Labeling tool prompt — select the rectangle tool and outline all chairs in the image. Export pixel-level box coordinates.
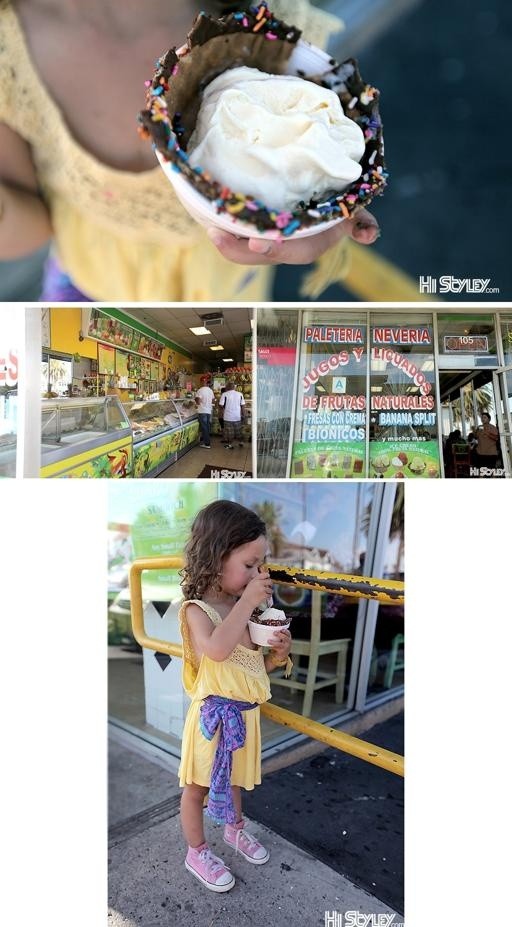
[263,589,351,719]
[452,442,471,477]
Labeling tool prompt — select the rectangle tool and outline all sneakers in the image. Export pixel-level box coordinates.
[222,819,270,865]
[185,841,236,893]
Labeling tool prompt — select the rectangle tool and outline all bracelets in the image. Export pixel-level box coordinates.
[266,649,289,667]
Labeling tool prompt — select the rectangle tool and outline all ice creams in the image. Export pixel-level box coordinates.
[250,608,292,626]
[138,6,387,238]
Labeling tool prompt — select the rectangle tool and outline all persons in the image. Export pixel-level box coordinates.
[1,0,384,302]
[216,387,231,444]
[177,498,294,894]
[194,375,217,449]
[472,412,500,478]
[442,429,478,475]
[218,383,247,450]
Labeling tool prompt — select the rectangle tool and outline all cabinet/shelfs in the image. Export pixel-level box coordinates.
[41,371,252,479]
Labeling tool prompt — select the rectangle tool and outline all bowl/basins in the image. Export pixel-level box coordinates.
[371,459,437,478]
